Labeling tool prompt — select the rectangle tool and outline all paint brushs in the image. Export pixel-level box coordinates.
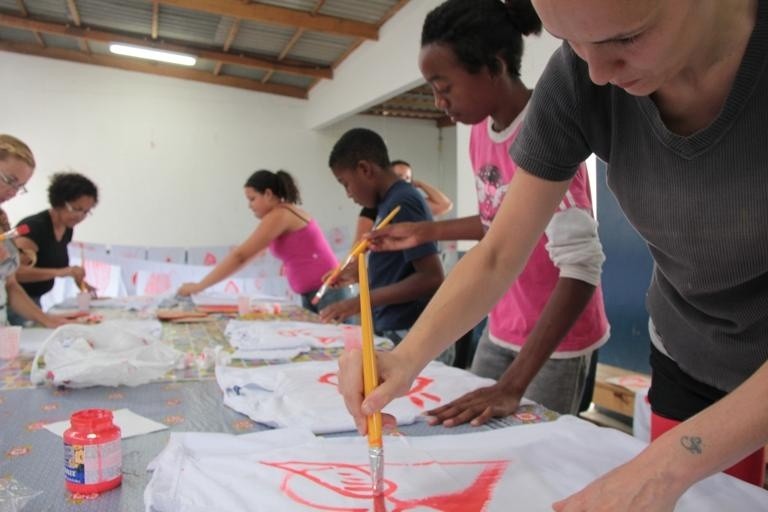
[0,223,30,242]
[357,253,388,512]
[80,249,87,293]
[311,204,402,306]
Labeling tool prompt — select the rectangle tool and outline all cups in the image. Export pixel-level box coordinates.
[2,325,21,360]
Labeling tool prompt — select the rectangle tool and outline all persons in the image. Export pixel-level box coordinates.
[336,0,768,512]
[359,0,611,431]
[6,171,100,328]
[0,133,97,329]
[317,127,456,367]
[350,159,452,259]
[175,168,357,326]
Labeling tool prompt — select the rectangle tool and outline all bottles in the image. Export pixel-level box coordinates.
[62,407,124,495]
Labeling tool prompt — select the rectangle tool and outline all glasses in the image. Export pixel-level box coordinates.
[0,169,28,196]
[65,202,93,218]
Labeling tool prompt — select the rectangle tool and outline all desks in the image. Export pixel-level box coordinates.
[0,293,768,512]
